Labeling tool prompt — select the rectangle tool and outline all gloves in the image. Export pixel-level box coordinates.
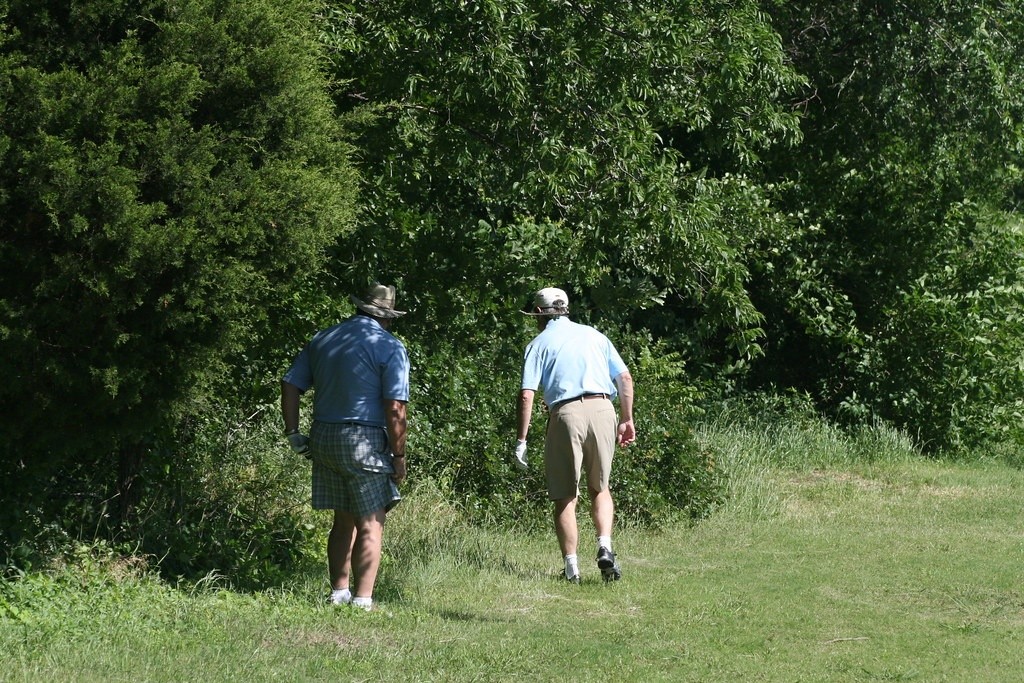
[514,438,528,470]
[285,427,312,460]
[391,454,407,487]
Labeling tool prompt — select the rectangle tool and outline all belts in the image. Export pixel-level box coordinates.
[560,393,611,402]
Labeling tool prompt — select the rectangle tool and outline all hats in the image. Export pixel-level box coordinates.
[351,280,407,318]
[531,287,569,313]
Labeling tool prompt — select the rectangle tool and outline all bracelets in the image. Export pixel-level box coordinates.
[391,454,405,458]
[283,427,299,436]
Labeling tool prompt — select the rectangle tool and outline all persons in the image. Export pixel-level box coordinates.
[514,287,636,583]
[280,284,411,617]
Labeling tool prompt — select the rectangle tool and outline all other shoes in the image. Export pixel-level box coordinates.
[348,602,393,620]
[330,593,354,608]
[595,546,621,583]
[558,569,581,586]
